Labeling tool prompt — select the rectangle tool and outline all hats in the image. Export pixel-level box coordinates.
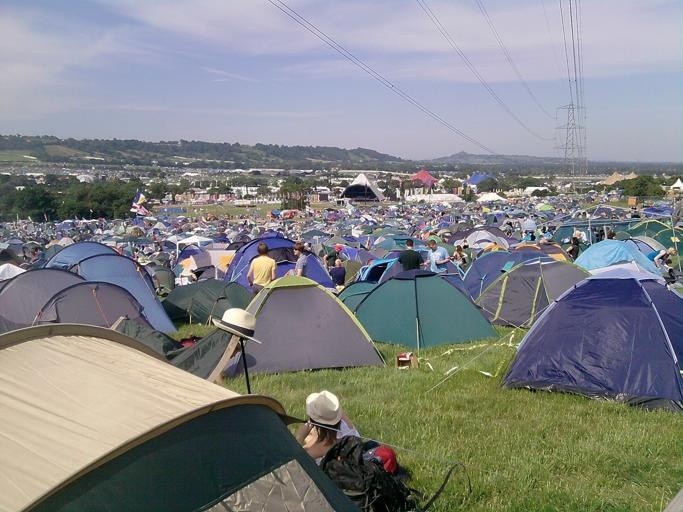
[211,307,262,345]
[305,390,342,426]
[335,245,342,251]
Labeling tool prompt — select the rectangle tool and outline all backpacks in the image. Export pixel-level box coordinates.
[319,435,421,512]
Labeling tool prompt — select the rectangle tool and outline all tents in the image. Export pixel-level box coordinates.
[0,188,682,415]
[0,323,366,512]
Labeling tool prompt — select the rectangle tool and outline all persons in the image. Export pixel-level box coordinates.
[296,390,361,467]
